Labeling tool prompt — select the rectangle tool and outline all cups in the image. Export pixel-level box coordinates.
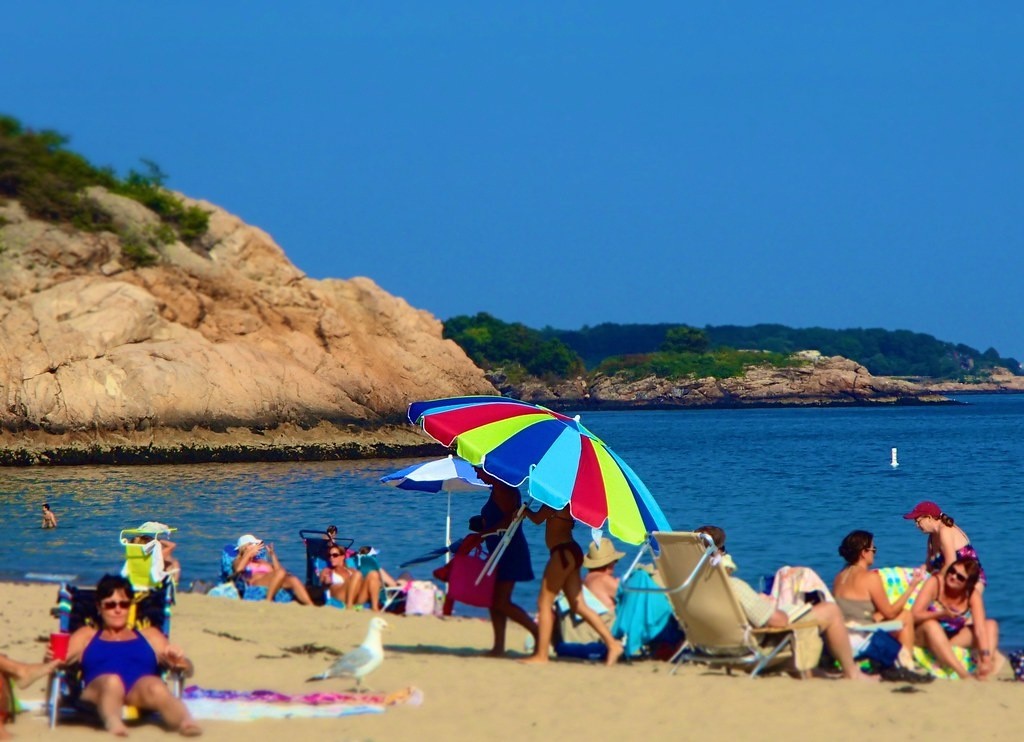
[50,633,72,663]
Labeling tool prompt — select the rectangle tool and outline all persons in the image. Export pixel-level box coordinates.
[360,546,407,588]
[904,502,987,624]
[0,654,60,688]
[520,502,624,667]
[831,530,936,683]
[43,573,202,736]
[911,555,998,681]
[468,464,539,657]
[583,538,627,610]
[233,533,316,606]
[322,526,338,546]
[131,534,180,583]
[41,503,57,528]
[329,547,382,613]
[692,526,881,683]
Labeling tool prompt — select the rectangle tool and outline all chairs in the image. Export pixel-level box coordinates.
[360,556,407,613]
[50,577,182,734]
[222,543,289,600]
[777,564,904,668]
[876,567,976,679]
[315,555,367,609]
[620,530,808,682]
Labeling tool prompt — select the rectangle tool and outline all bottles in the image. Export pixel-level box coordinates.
[436,590,446,616]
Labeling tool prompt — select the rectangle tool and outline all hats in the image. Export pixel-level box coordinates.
[583,536,626,570]
[902,501,942,520]
[359,547,382,560]
[234,533,263,552]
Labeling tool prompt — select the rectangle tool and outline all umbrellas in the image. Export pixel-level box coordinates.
[399,538,466,569]
[406,395,672,587]
[378,454,492,595]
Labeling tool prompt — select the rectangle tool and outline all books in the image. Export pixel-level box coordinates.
[778,600,813,625]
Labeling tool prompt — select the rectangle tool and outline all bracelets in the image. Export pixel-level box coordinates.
[979,650,989,656]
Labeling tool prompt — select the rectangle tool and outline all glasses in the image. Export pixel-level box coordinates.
[718,546,726,552]
[327,553,344,558]
[948,567,971,585]
[865,547,877,553]
[104,601,136,610]
